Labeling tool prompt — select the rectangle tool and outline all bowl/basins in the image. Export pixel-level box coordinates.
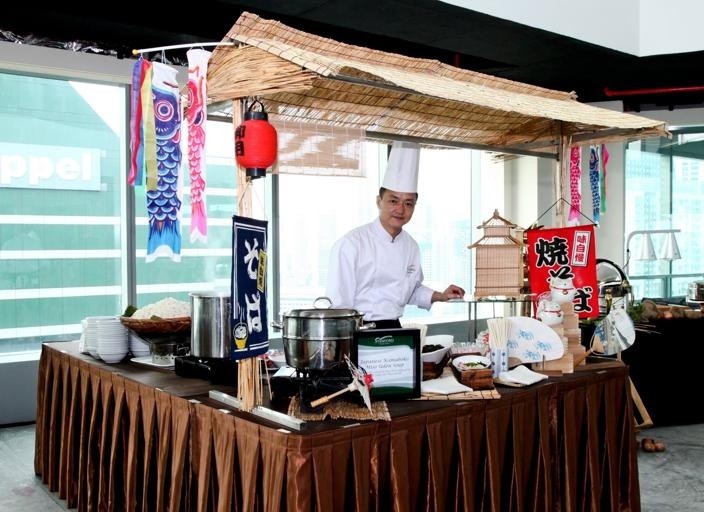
[189,290,237,359]
[449,341,490,360]
[117,315,191,341]
[85,312,151,363]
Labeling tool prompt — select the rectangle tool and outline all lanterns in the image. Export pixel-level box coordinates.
[233,109,281,181]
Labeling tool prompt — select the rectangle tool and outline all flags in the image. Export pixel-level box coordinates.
[527,225,598,319]
[228,215,269,362]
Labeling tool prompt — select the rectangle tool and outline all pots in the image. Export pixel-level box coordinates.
[270,297,376,373]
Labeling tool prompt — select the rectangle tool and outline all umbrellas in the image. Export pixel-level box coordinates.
[309,350,376,419]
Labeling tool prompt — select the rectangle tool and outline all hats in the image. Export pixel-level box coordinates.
[381,141,420,194]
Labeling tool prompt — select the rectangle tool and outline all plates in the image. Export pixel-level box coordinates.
[130,355,181,371]
[490,317,565,363]
[452,356,490,372]
[422,335,454,363]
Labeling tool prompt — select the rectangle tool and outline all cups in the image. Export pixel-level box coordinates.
[490,347,509,377]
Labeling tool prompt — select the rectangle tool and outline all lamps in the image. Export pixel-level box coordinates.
[626,228,682,278]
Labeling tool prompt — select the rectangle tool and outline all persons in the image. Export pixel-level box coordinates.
[335,185,468,330]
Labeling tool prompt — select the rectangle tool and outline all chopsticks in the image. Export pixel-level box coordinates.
[487,318,513,348]
